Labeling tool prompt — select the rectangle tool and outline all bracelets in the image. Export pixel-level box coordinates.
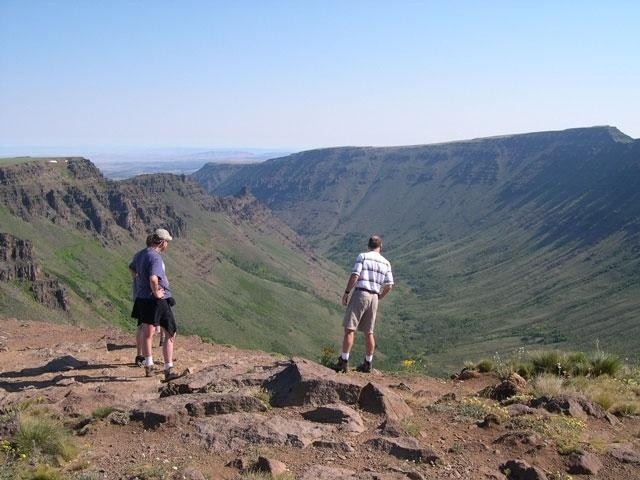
[345,289,350,294]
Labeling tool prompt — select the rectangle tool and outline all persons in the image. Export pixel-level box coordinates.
[336,235,394,372]
[128,229,186,381]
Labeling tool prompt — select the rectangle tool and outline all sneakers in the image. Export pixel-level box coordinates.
[160,340,165,346]
[164,367,181,380]
[135,355,164,377]
[357,361,371,373]
[154,229,173,241]
[331,357,349,373]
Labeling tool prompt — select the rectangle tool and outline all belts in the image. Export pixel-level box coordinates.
[355,287,376,294]
